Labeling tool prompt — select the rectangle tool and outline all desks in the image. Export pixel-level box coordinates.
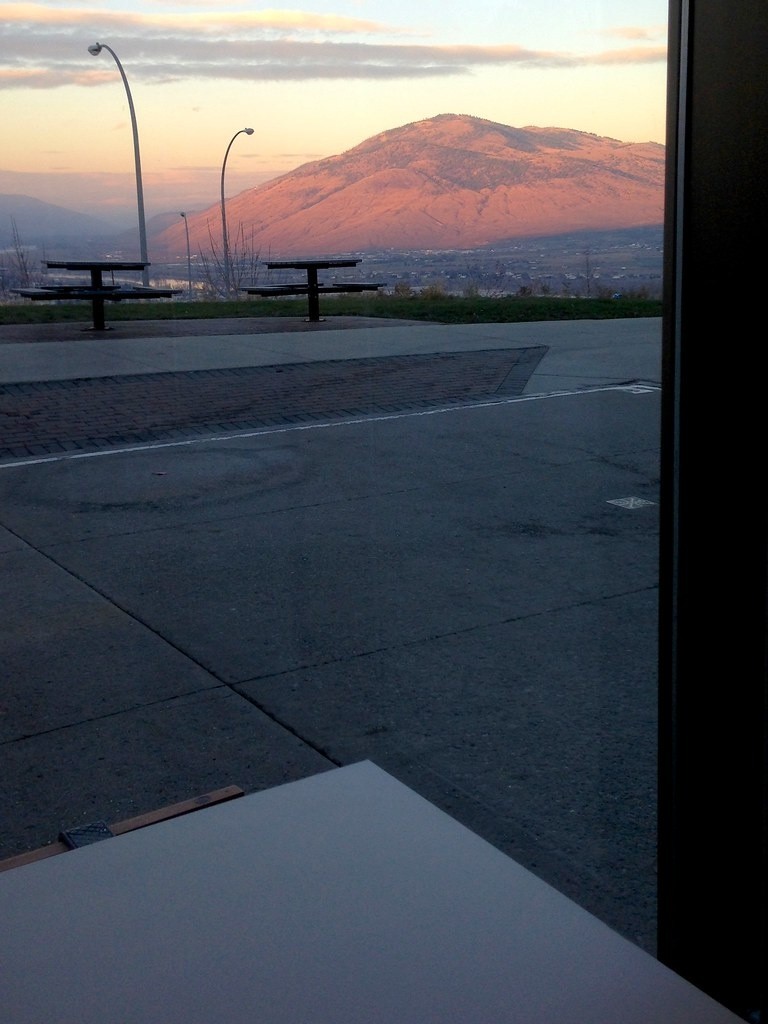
[20,260,173,331]
[245,259,379,322]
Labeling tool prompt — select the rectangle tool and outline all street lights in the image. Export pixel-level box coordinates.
[221,128,254,301]
[180,212,192,302]
[88,42,150,303]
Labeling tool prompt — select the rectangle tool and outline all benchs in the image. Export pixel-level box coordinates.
[333,281,387,288]
[258,282,325,289]
[10,288,57,295]
[133,285,183,295]
[237,285,288,292]
[37,285,121,291]
